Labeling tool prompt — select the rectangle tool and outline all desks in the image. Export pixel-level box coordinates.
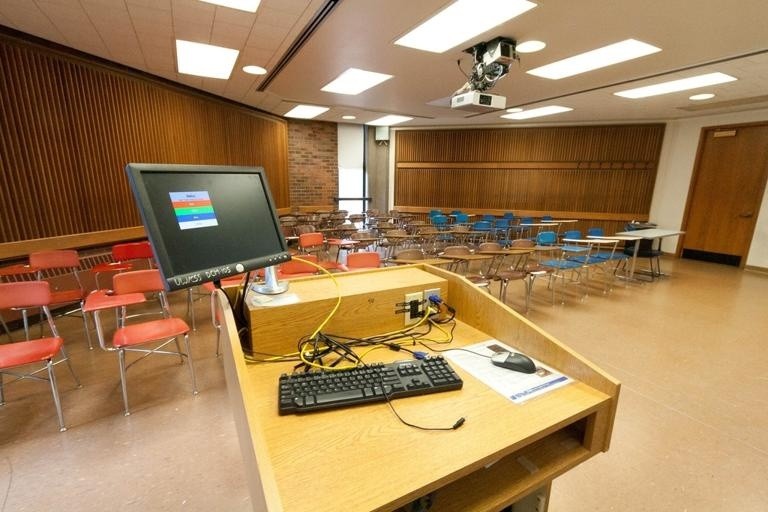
[90,260,134,273]
[1,263,40,276]
[83,289,147,312]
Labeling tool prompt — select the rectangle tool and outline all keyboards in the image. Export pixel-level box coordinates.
[279,356,463,415]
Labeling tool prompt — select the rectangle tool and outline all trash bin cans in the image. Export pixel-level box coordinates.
[623,222,658,257]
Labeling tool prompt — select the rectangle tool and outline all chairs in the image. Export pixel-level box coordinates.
[187,203,685,316]
[1,280,84,434]
[11,248,94,351]
[94,269,200,417]
[94,240,156,291]
[203,279,248,356]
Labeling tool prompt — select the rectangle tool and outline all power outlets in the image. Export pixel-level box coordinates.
[423,288,441,321]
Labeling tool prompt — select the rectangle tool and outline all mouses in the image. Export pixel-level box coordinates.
[492,352,536,374]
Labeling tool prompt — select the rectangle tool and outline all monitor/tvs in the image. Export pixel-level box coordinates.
[125,163,291,293]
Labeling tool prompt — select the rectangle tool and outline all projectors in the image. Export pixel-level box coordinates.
[451,92,506,113]
